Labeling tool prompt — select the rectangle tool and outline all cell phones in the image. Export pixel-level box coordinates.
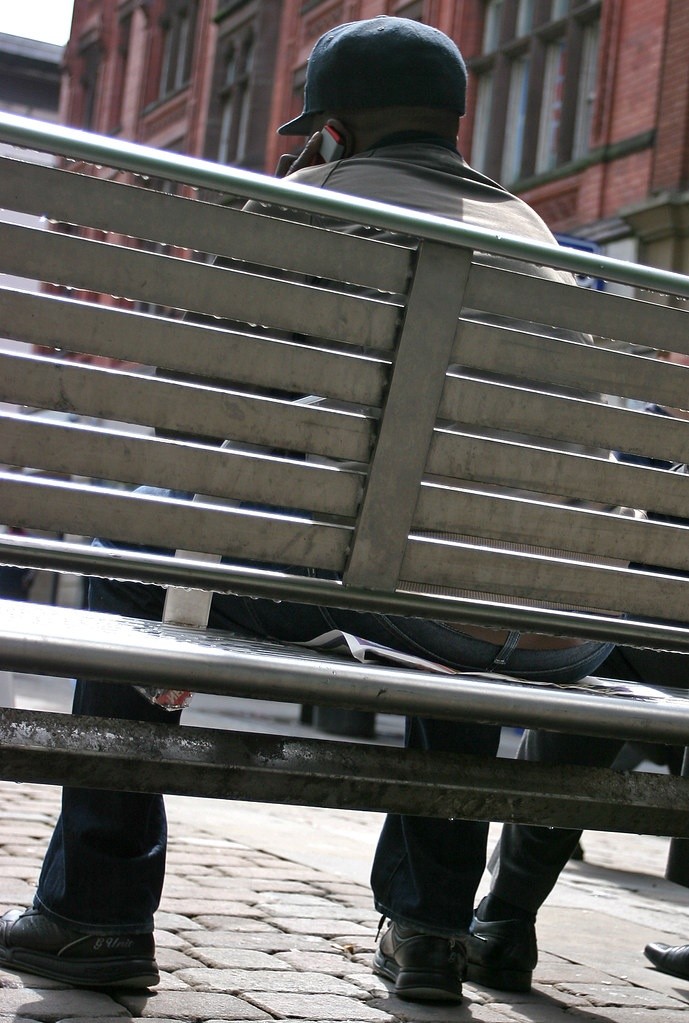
[311,126,344,167]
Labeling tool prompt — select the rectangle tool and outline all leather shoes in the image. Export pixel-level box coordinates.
[644,940,689,977]
[459,895,538,988]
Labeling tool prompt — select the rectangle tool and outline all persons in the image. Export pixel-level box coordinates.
[461,462,689,991]
[0,15,617,1005]
[615,351,688,469]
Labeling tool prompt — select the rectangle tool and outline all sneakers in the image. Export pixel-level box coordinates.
[374,911,464,1005]
[0,902,161,987]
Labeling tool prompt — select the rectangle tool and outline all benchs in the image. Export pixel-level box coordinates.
[0,114,689,837]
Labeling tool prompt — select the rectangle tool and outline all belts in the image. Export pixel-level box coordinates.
[439,617,590,650]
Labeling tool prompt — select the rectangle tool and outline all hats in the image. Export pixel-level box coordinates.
[275,15,467,135]
